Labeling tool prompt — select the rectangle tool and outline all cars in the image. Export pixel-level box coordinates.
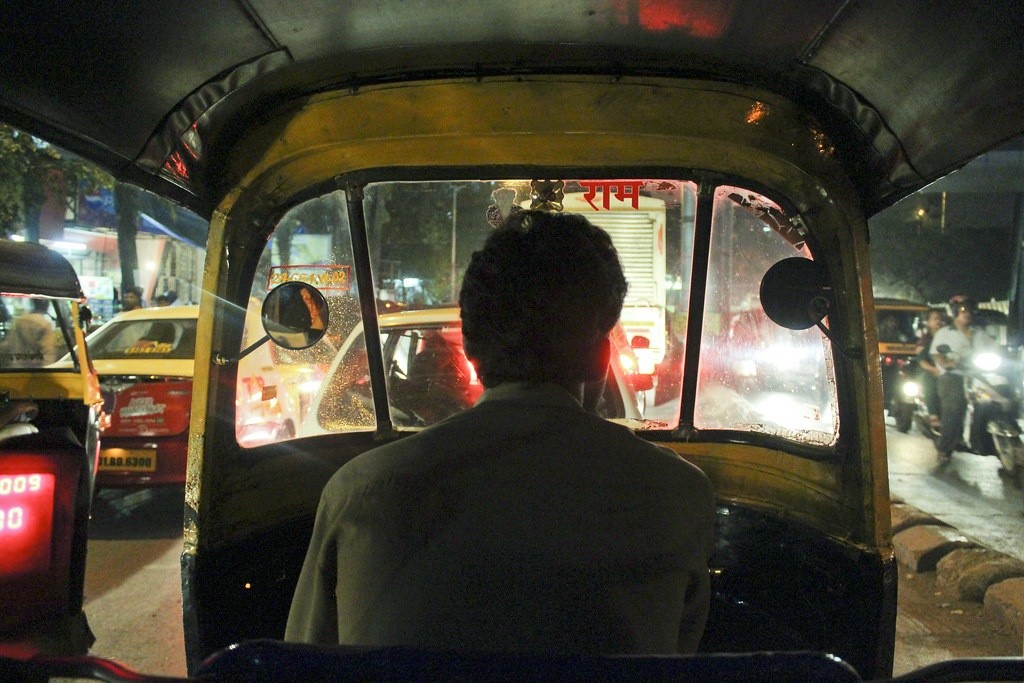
[43,304,323,486]
[311,307,641,432]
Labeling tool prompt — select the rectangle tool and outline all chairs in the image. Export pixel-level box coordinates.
[138,323,178,344]
[406,348,472,392]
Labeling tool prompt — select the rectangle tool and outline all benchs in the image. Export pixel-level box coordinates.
[201,637,860,683]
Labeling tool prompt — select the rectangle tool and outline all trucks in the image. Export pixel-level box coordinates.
[515,191,674,416]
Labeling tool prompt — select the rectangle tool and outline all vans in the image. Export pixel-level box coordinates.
[1,234,99,659]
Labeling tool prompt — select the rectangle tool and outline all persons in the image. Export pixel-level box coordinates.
[284,208,713,660]
[0,287,184,366]
[874,293,1005,470]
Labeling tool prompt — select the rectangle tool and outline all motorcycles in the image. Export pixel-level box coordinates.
[868,297,939,433]
[914,330,1024,480]
